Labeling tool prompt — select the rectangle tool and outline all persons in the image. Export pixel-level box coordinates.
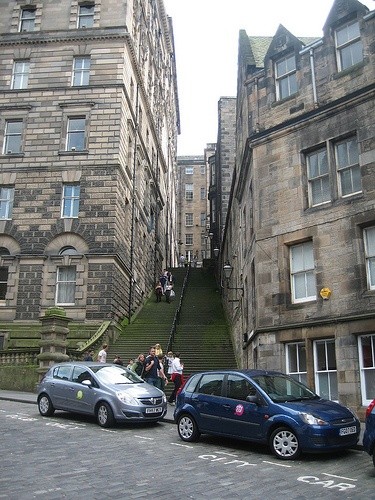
[179,254,188,268]
[193,254,197,267]
[155,269,176,303]
[84,344,185,406]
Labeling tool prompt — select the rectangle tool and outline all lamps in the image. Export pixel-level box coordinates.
[224,264,232,279]
[214,246,219,257]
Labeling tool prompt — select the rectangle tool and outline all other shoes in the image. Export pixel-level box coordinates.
[167,402,176,406]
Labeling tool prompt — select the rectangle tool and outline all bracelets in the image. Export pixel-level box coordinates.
[165,377,167,380]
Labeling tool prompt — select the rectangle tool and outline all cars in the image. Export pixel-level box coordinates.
[36,361,168,428]
[173,369,362,460]
[362,399,375,465]
